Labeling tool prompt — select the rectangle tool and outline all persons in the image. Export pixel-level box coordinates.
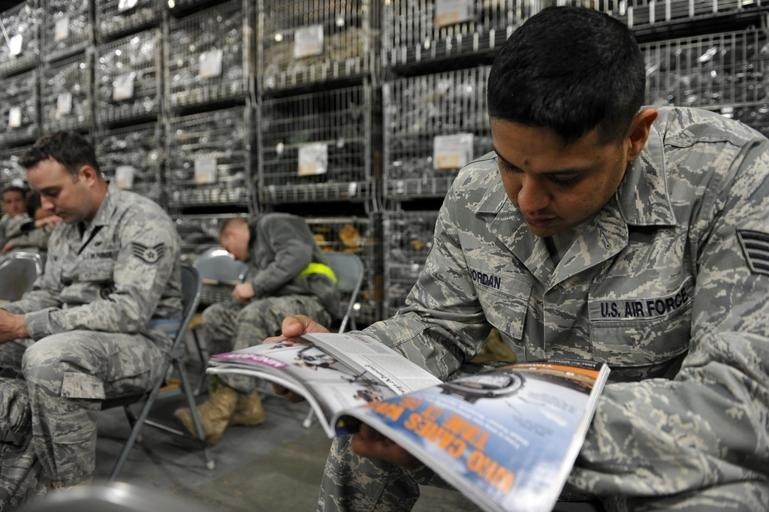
[171,210,342,449]
[4,193,63,261]
[0,129,186,511]
[258,0,769,512]
[1,185,32,243]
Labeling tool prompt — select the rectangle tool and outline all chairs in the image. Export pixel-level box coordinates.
[102,265,214,482]
[256,252,365,428]
[186,248,245,397]
[0,251,44,301]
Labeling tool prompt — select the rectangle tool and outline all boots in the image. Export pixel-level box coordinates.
[172,382,266,447]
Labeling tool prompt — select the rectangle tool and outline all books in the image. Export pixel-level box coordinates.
[202,326,614,512]
